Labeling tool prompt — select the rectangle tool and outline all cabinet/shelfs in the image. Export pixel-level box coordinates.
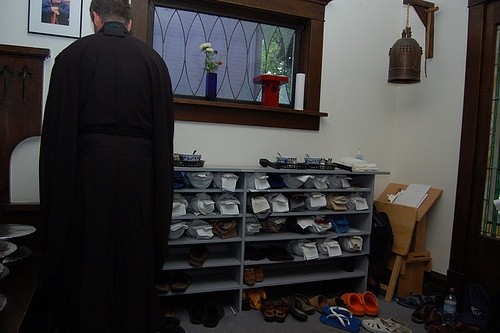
[0,43,51,333]
[160,165,392,314]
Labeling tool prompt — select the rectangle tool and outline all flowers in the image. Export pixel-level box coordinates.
[199,42,223,73]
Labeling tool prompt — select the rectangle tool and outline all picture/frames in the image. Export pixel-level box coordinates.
[27,0,83,40]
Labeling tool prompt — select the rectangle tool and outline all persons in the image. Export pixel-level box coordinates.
[39,0,175,333]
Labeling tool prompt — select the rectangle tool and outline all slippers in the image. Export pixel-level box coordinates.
[308,295,350,313]
[341,291,379,316]
[261,302,288,323]
[319,306,361,332]
[282,293,315,321]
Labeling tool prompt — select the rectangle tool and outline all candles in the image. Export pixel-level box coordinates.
[294,73,306,110]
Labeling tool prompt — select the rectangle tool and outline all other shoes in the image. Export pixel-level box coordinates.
[157,279,169,294]
[188,301,225,328]
[411,303,430,323]
[424,306,442,331]
[244,267,255,286]
[189,246,208,267]
[254,268,265,283]
[395,293,433,308]
[170,273,192,293]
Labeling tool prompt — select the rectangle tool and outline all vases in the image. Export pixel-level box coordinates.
[205,72,218,98]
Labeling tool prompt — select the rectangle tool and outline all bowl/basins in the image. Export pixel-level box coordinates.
[303,158,321,164]
[276,156,297,164]
[179,154,202,161]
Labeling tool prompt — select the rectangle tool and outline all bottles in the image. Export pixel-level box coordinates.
[319,158,326,169]
[327,158,333,169]
[444,287,457,322]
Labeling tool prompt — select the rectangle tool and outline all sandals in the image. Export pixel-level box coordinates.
[362,318,412,333]
